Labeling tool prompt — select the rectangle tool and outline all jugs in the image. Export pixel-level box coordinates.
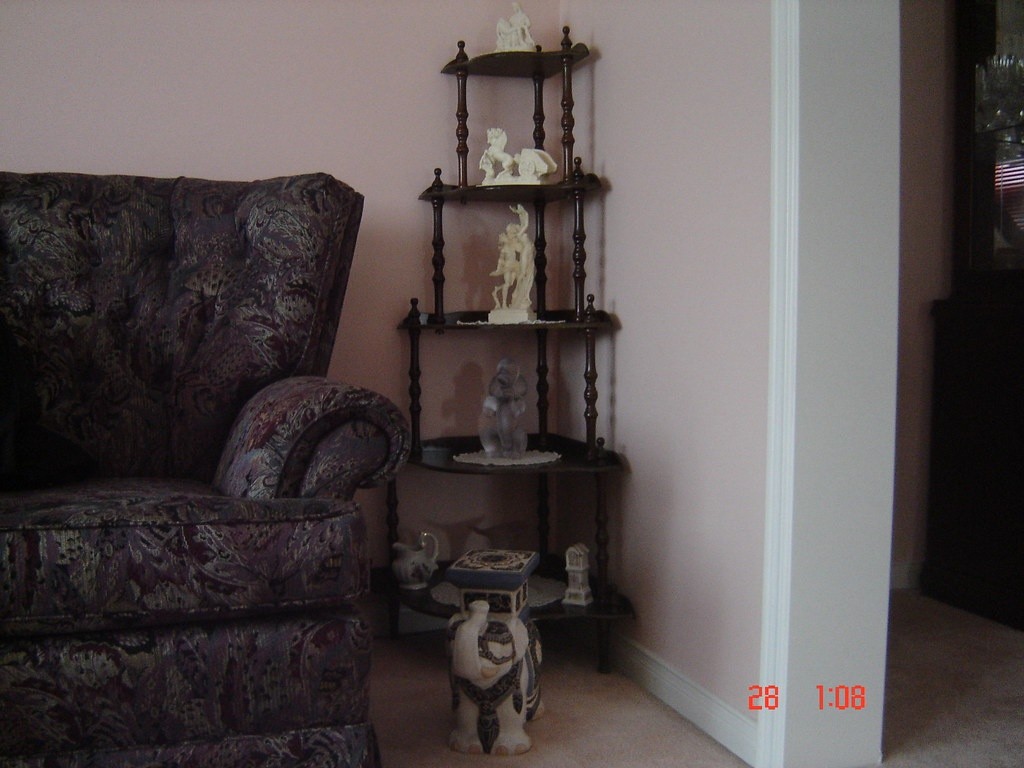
[391,531,439,591]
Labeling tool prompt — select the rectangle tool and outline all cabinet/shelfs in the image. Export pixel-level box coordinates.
[919,0,1024,633]
[375,23,639,674]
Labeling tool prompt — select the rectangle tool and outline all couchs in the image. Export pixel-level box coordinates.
[0,171,410,768]
